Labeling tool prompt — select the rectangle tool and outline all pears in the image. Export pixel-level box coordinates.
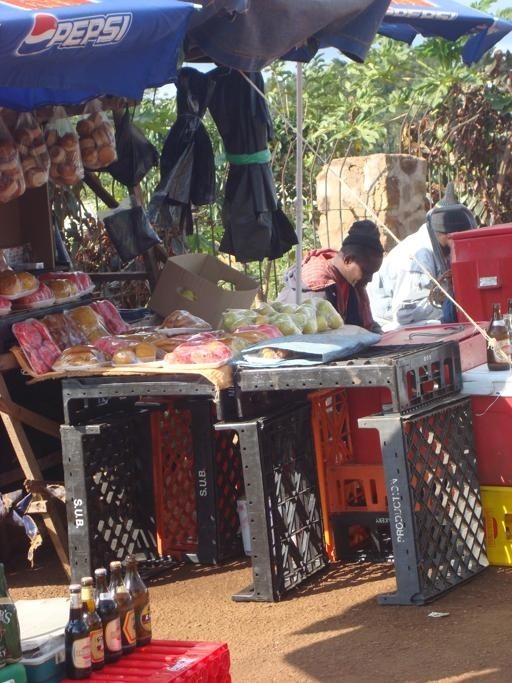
[181,288,195,301]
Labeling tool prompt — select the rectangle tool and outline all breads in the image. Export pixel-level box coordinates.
[0,111,117,203]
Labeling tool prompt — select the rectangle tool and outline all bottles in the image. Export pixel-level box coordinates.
[64,553,153,680]
[485,302,511,370]
[0,563,22,667]
[504,299,511,342]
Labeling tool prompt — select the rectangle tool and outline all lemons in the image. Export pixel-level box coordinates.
[112,350,132,364]
[135,343,156,362]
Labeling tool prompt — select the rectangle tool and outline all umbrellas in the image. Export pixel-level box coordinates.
[0,0,512,306]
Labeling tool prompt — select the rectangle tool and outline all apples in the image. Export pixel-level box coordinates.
[221,296,344,335]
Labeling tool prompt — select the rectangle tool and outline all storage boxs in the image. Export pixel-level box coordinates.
[149,253,262,328]
[459,362,512,486]
[14,598,72,683]
[448,222,512,323]
[346,319,489,464]
[237,494,297,557]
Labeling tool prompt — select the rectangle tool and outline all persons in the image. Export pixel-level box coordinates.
[366,204,479,333]
[275,219,385,336]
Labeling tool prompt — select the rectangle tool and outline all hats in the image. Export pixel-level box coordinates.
[425,179,477,234]
